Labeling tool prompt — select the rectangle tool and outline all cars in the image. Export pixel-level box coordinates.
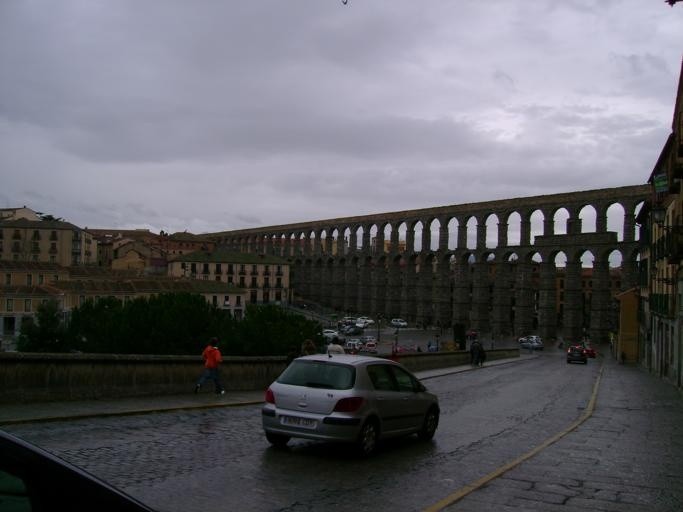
[262,350,440,453]
[317,316,378,355]
[575,341,596,358]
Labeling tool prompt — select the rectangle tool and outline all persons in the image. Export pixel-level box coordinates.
[192,337,226,395]
[326,336,345,354]
[286,345,299,367]
[303,340,313,356]
[558,343,566,368]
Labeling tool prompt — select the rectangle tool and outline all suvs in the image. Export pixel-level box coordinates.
[518,335,545,350]
[567,346,587,365]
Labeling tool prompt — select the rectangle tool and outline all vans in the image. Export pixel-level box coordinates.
[391,318,408,328]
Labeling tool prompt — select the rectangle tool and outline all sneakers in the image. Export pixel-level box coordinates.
[216,389,226,397]
[192,383,200,395]
